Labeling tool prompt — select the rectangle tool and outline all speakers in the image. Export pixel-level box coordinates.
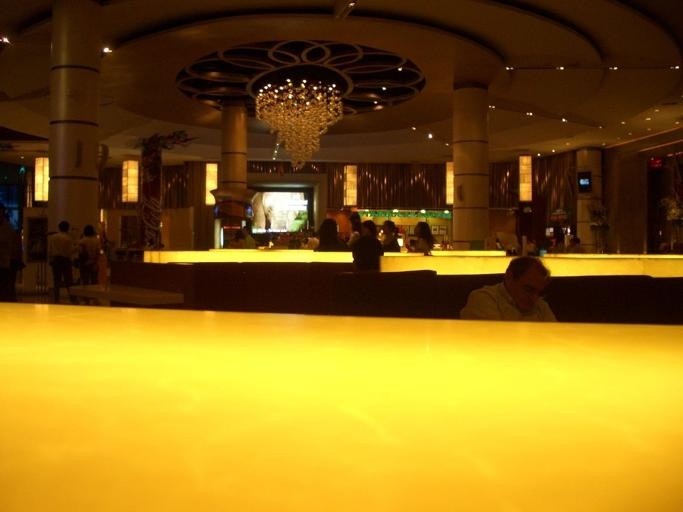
[25,217,48,263]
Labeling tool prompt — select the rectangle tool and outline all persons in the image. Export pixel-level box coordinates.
[547,226,565,252]
[4,211,27,301]
[0,202,19,302]
[45,220,75,304]
[75,225,100,305]
[460,255,556,321]
[230,217,433,298]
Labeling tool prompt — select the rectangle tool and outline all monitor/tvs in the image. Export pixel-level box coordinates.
[577,171,593,193]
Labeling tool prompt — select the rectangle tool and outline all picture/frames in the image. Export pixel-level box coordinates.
[431,225,439,236]
[439,225,447,236]
[376,224,415,236]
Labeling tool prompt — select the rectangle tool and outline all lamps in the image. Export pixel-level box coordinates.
[518,155,532,202]
[344,162,357,206]
[122,160,139,203]
[254,76,345,172]
[34,156,49,202]
[204,162,218,206]
[445,161,454,205]
